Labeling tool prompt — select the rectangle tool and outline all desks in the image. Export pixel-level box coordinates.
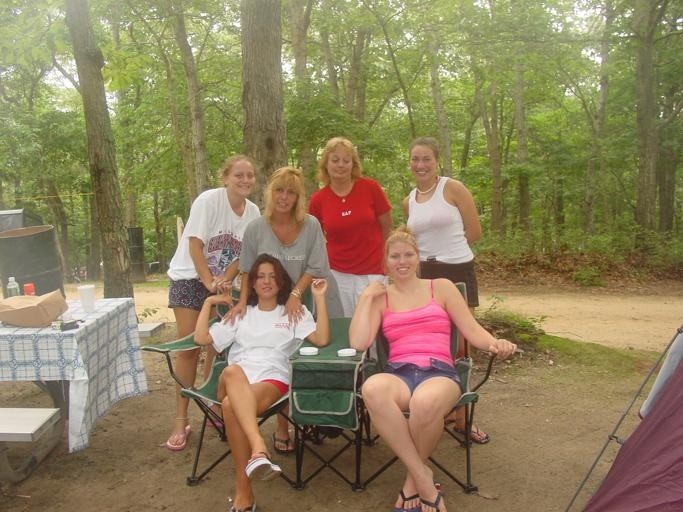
[290,317,370,367]
[1,297,136,441]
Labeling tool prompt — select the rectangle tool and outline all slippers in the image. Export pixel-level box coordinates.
[453,424,489,444]
[167,424,192,452]
[421,479,445,512]
[229,501,256,512]
[272,431,296,454]
[245,452,282,481]
[393,478,420,512]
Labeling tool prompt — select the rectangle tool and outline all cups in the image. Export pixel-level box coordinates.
[77,284,96,313]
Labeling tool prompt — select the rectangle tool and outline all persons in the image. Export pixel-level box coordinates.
[398,136,491,444]
[348,223,517,512]
[222,165,330,328]
[167,154,261,451]
[308,137,392,360]
[193,253,329,512]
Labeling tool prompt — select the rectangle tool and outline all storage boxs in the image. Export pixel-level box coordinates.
[0,289,69,329]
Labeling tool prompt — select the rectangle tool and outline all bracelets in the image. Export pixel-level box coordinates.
[289,288,302,298]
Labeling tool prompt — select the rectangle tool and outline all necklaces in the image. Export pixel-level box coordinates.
[417,176,439,194]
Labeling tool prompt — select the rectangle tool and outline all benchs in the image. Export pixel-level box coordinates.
[134,322,166,338]
[0,407,61,484]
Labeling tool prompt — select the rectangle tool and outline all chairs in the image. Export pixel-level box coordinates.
[365,281,513,495]
[142,317,296,488]
[288,361,368,490]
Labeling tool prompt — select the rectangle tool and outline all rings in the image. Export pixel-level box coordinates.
[229,313,232,315]
[297,310,300,313]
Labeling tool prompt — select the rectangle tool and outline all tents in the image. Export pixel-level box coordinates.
[561,325,682,510]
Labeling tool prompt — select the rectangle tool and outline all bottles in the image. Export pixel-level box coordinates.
[6,277,36,297]
